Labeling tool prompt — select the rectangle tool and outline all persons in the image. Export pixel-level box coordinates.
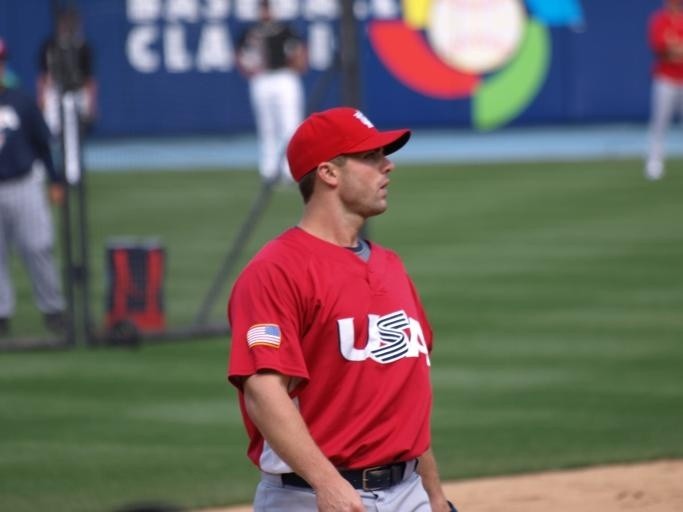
[0,39,70,339]
[33,0,100,194]
[226,103,456,512]
[237,0,309,188]
[644,0,682,181]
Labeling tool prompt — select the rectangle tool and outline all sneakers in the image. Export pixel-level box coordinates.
[0,314,94,336]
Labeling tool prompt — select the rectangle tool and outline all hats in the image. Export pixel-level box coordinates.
[286,106,409,182]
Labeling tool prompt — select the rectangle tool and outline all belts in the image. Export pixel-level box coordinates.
[281,457,418,492]
